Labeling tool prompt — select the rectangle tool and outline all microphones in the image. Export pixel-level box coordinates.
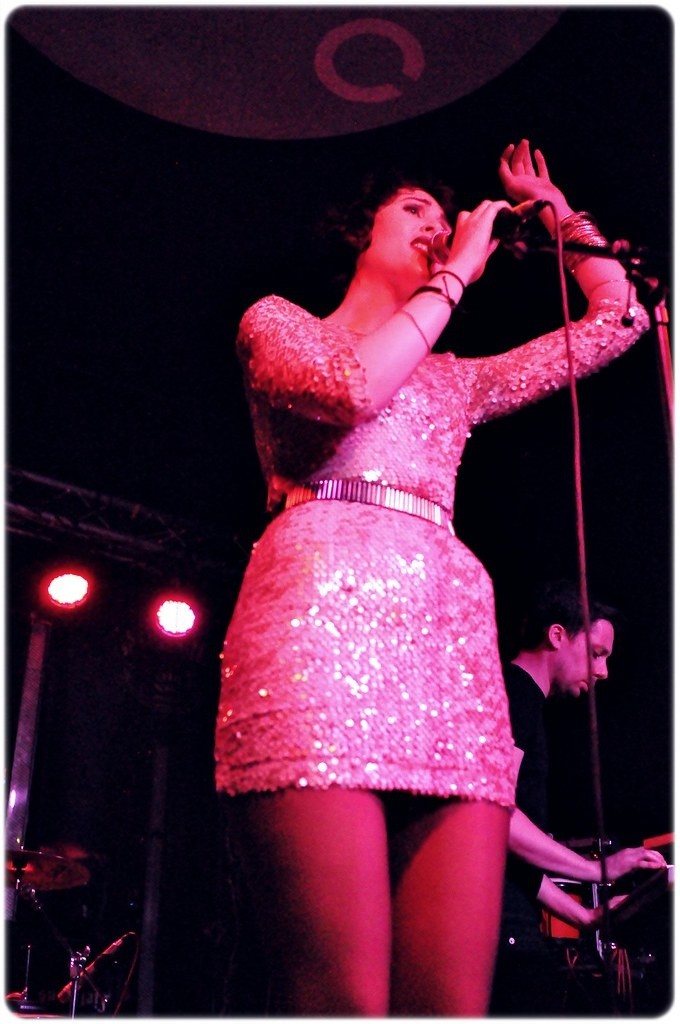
[58,931,134,1003]
[428,198,549,265]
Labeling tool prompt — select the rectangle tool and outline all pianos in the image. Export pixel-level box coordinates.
[597,864,674,948]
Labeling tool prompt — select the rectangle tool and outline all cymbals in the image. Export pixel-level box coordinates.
[5,848,89,890]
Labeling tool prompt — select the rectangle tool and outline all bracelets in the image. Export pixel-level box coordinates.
[585,280,633,298]
[550,212,609,272]
[427,270,465,290]
[393,308,432,353]
[404,276,459,313]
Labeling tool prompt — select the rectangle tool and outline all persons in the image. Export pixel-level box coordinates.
[500,604,668,1019]
[215,139,649,1018]
